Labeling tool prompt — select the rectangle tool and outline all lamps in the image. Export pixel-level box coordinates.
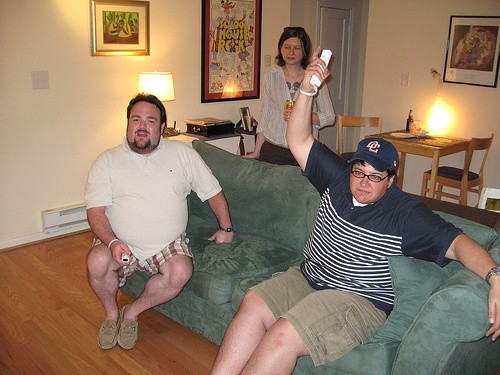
[137,72,179,136]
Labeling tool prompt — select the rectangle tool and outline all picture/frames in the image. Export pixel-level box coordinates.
[90,0,151,56]
[239,106,254,130]
[443,15,499,88]
[201,0,263,103]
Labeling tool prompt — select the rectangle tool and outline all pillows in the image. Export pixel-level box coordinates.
[367,254,450,344]
[444,221,497,276]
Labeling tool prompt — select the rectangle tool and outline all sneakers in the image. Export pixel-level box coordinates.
[97,310,120,349]
[118,304,139,350]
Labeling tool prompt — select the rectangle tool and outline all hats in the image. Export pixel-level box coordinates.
[349,138,399,175]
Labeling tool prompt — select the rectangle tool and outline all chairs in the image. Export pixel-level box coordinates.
[337,114,383,156]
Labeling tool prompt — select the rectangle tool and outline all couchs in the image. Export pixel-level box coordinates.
[116,141,498,375]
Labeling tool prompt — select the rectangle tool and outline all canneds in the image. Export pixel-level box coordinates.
[284,99,294,120]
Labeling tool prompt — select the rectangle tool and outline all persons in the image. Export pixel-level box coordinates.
[209,45,500,375]
[240,26,336,165]
[84,92,234,350]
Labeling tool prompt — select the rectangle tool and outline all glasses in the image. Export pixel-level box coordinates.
[350,164,393,182]
[283,27,304,32]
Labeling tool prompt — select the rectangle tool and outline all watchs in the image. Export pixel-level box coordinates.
[485,266,500,284]
[219,227,233,232]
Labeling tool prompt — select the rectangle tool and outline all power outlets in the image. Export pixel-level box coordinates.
[30,71,48,91]
